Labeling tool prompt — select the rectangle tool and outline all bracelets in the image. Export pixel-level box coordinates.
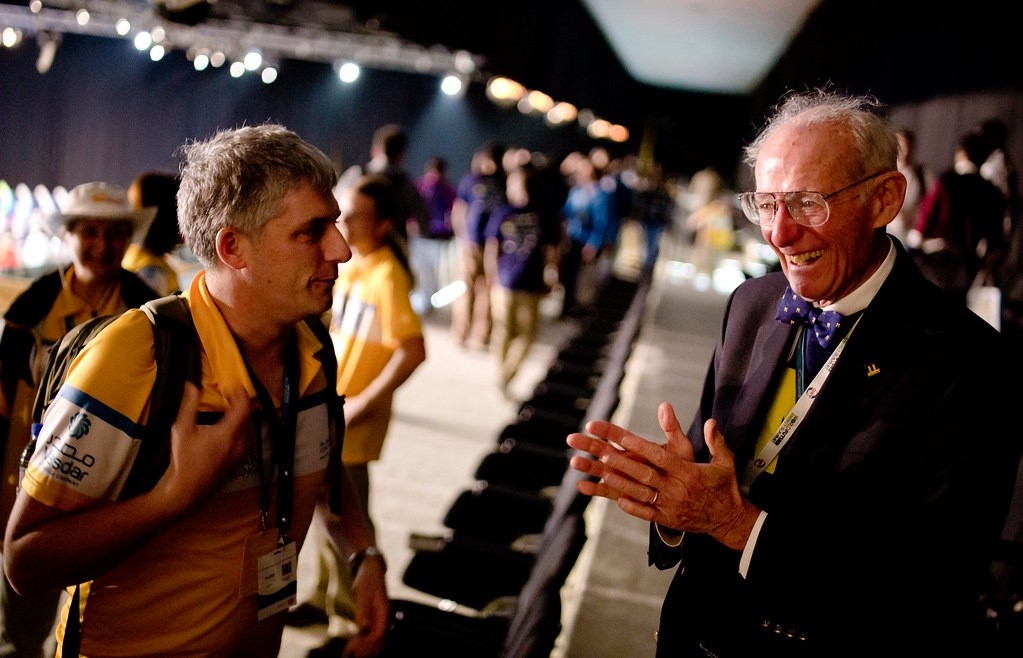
[347,547,388,581]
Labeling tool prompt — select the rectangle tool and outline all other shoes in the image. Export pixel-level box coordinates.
[284,601,329,627]
[307,635,349,658]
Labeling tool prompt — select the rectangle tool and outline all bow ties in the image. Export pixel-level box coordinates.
[775,285,843,349]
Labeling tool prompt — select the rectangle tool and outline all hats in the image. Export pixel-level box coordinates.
[48,181,157,245]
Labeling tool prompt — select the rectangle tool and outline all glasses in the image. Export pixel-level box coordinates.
[737,173,880,227]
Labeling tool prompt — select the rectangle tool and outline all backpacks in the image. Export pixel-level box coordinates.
[13,292,201,532]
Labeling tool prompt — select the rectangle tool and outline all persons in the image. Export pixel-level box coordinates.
[416,153,608,398]
[0,126,431,658]
[565,97,1023,658]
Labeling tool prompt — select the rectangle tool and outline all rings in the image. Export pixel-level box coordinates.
[650,490,659,506]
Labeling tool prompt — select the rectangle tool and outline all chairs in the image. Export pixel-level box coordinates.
[300,251,655,658]
